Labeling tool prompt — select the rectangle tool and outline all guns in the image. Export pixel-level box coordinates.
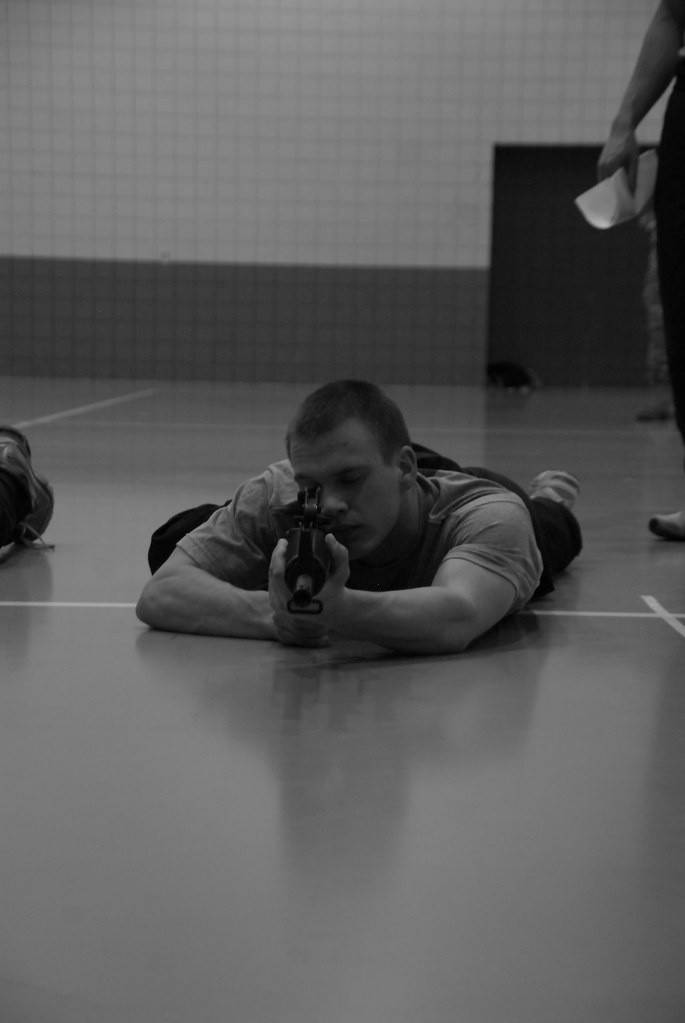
[279,484,332,614]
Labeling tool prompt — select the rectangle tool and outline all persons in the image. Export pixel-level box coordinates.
[0,424,53,549]
[593,0,685,540]
[137,379,584,656]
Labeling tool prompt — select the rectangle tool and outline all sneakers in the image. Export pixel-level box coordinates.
[0,425,54,551]
[526,470,582,509]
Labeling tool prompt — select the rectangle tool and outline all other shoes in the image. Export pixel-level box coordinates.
[649,511,685,541]
[636,407,672,421]
